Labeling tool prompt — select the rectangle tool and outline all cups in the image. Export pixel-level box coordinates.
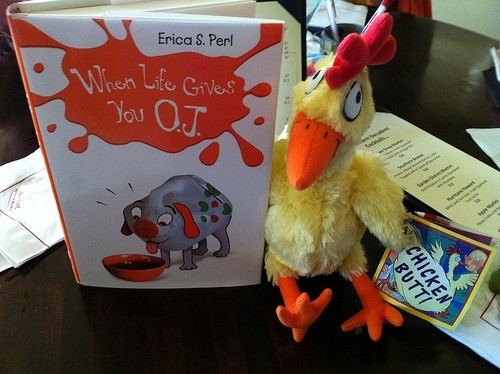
[320,23,368,58]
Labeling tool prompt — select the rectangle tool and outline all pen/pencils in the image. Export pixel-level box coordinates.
[325,0,340,43]
[415,211,500,244]
[360,0,391,37]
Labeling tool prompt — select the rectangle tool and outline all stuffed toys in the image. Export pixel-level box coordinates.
[264,12,408,342]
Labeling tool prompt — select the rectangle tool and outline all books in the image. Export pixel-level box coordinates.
[6,1,287,290]
